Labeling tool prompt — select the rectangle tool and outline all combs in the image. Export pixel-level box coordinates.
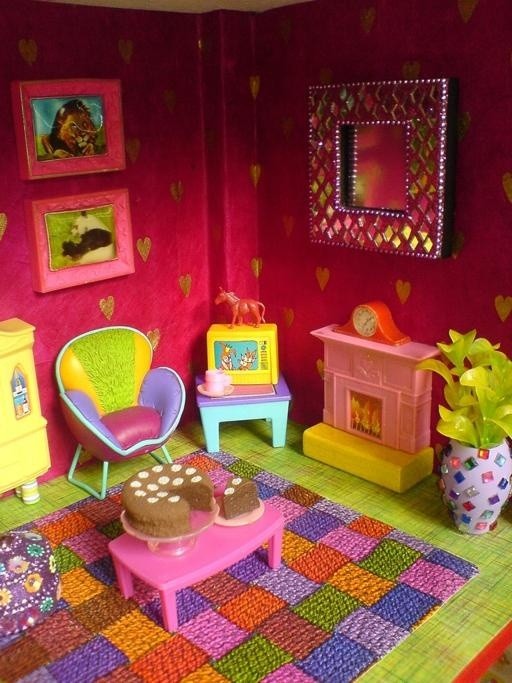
[214,287,267,327]
